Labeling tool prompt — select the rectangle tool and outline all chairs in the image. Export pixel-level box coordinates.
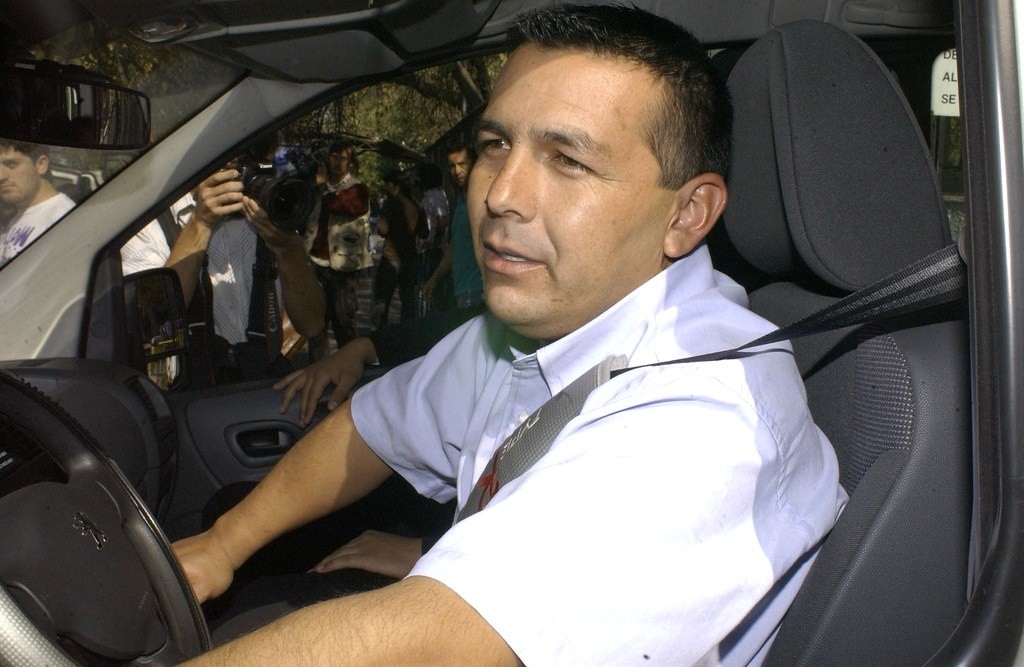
[703,19,977,667]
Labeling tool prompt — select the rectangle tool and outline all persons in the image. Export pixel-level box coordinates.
[170,0,848,667]
[1,138,488,612]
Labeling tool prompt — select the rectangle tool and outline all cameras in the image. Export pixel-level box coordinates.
[229,151,320,230]
